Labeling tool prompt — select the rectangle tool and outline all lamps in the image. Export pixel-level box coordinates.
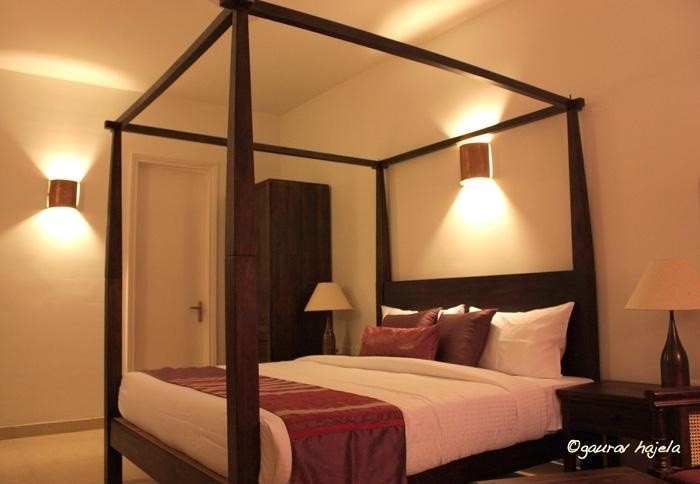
[300,281,354,359]
[47,177,79,215]
[457,139,492,184]
[625,256,700,385]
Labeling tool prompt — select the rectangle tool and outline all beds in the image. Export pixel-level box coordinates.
[102,0,599,484]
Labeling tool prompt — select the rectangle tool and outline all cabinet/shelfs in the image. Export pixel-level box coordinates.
[253,178,334,361]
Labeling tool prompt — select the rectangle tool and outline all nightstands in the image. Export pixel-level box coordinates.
[553,374,700,473]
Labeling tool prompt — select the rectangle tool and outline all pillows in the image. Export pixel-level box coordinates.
[379,303,467,319]
[435,306,502,368]
[377,306,442,326]
[452,298,575,379]
[354,325,440,359]
